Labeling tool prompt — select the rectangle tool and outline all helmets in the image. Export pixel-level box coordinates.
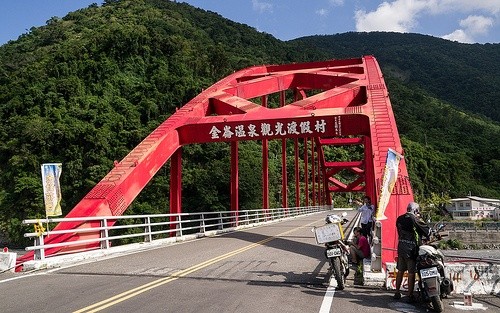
[407,203,420,215]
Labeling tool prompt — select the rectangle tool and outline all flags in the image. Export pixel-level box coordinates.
[42,163,62,216]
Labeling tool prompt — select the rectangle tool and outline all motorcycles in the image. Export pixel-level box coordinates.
[416,218,454,313]
[324,211,351,290]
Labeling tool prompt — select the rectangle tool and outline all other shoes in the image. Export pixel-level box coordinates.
[406,295,420,303]
[392,292,402,301]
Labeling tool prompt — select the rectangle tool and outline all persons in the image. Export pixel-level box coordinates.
[394,202,428,299]
[346,195,377,268]
